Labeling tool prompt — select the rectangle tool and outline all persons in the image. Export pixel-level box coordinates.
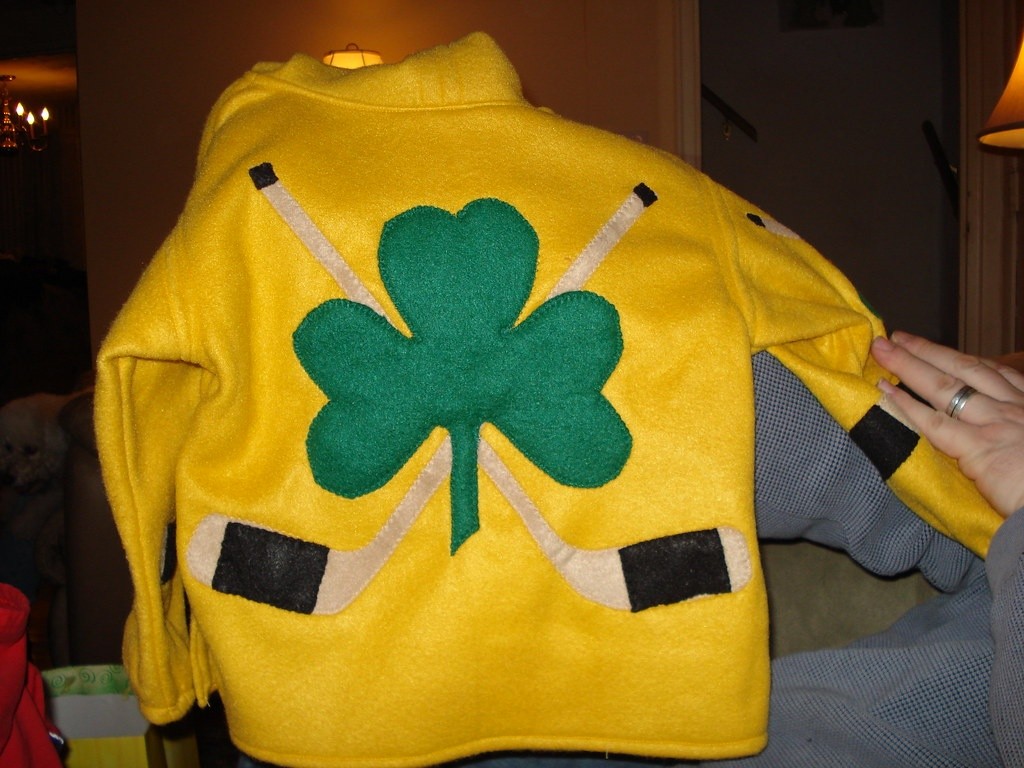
[492,332,1024,768]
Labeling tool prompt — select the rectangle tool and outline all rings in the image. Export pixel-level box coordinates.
[946,385,978,420]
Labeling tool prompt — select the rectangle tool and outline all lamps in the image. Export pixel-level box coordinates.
[13,101,52,152]
